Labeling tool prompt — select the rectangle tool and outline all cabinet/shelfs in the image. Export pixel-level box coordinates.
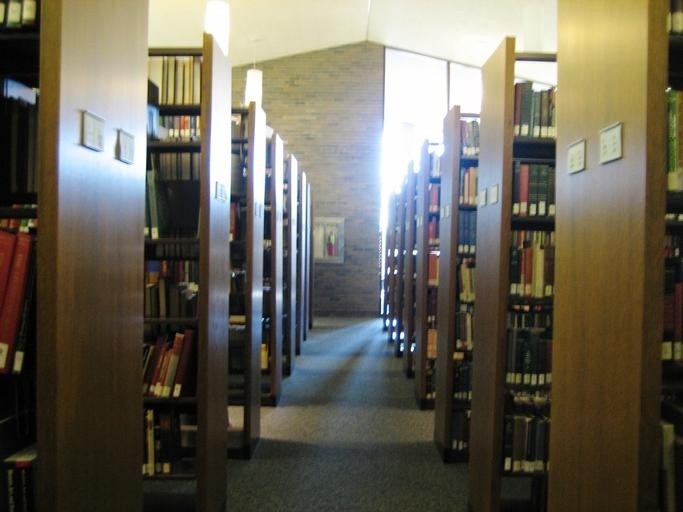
[385,0,683,512]
[0,0,310,512]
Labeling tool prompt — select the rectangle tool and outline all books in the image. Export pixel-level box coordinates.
[0,2,42,512]
[657,0,683,511]
[424,120,481,465]
[499,81,554,510]
[260,143,291,398]
[226,111,249,450]
[143,55,200,478]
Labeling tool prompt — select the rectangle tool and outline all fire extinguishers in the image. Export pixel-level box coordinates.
[327,232,337,258]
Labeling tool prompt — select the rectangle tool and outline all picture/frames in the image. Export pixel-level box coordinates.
[313,217,344,265]
